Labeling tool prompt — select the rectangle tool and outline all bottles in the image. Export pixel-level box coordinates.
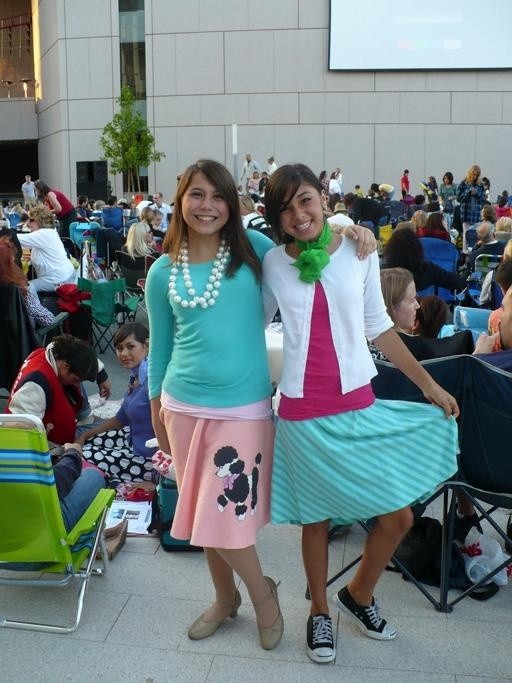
[469,273,480,296]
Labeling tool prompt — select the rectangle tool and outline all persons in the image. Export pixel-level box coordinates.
[0,440,129,575]
[79,324,169,488]
[4,334,111,449]
[159,162,460,663]
[147,158,378,651]
[2,153,512,421]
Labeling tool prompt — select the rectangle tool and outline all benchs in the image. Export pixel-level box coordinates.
[305,355,512,612]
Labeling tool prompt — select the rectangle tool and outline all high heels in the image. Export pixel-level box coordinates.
[96,520,129,561]
[252,576,284,650]
[188,589,242,640]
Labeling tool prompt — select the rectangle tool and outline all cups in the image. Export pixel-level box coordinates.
[451,229,458,240]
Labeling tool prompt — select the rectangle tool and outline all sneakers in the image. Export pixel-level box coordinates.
[332,586,399,640]
[306,612,336,663]
[447,503,483,550]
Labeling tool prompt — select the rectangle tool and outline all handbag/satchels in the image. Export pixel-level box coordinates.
[385,516,499,601]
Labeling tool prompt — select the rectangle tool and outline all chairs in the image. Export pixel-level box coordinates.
[1,414,115,633]
[358,197,512,349]
[1,208,166,354]
